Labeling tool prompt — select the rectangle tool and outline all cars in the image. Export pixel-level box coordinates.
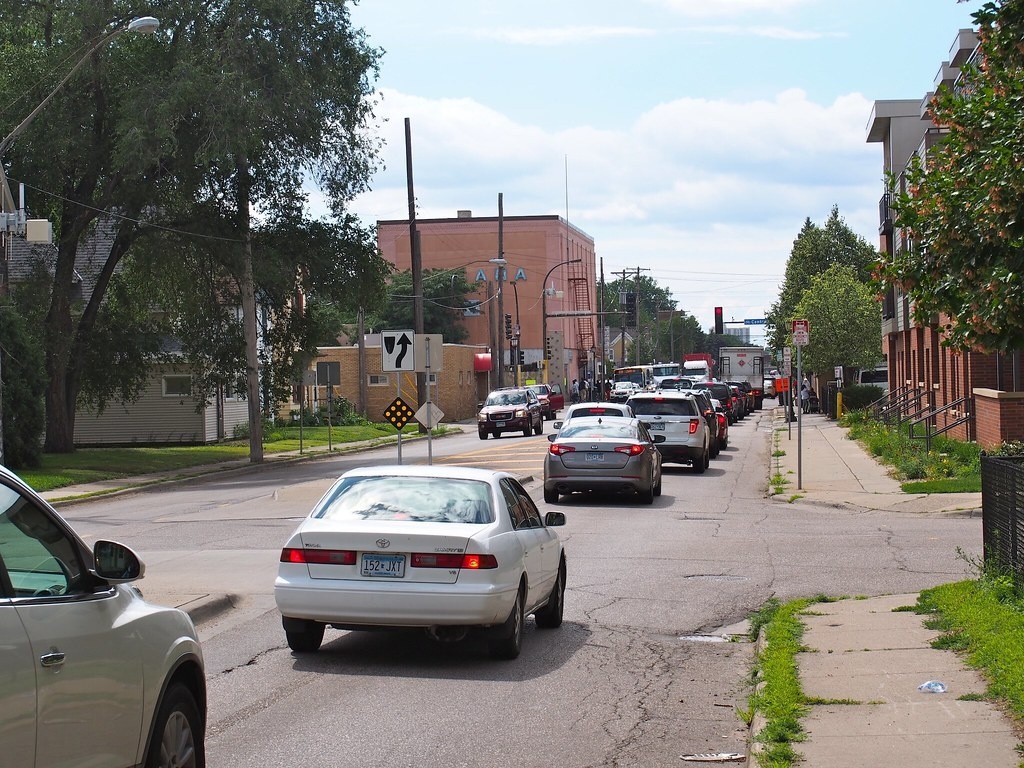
[543,417,664,506]
[567,402,637,422]
[614,347,780,473]
[0,457,209,768]
[478,385,539,440]
[277,464,575,664]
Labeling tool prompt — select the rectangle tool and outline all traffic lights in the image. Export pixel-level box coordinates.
[510,350,526,369]
[546,343,554,359]
[504,314,524,346]
[625,293,640,331]
[709,306,726,336]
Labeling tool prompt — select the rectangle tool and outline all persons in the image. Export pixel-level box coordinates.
[793,378,818,414]
[572,378,612,404]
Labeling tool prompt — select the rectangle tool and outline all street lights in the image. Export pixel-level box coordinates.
[541,258,584,395]
[415,257,509,424]
[1,13,167,162]
[511,278,521,389]
